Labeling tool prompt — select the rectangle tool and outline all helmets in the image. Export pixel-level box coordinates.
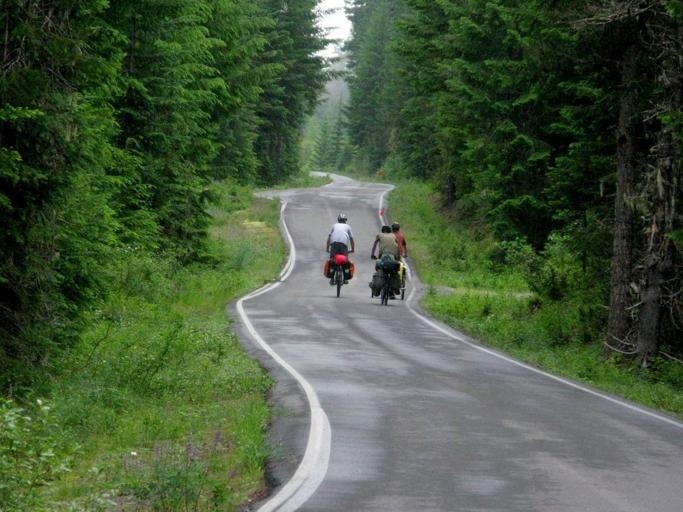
[337,214,347,221]
[392,222,400,229]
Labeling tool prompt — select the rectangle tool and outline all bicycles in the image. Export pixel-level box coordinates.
[325,250,354,298]
[371,255,409,306]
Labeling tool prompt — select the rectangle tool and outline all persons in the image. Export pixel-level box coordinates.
[326,213,355,285]
[391,222,407,258]
[370,225,403,300]
